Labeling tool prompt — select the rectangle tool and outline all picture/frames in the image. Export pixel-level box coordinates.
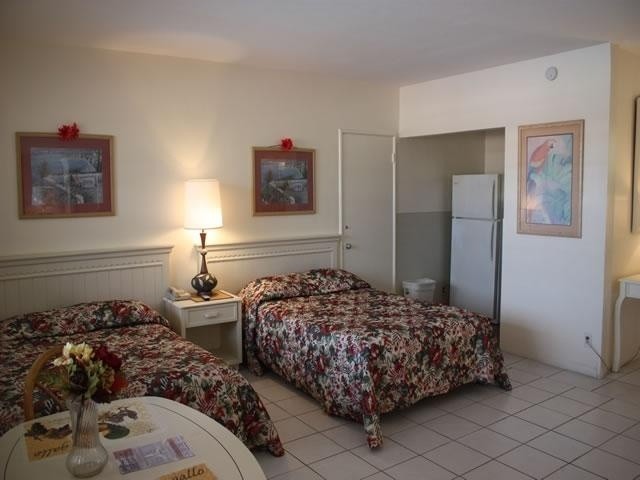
[517,119,584,238]
[14,130,115,219]
[630,96,640,235]
[251,145,317,216]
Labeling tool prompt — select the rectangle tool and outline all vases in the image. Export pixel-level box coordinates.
[65,402,109,477]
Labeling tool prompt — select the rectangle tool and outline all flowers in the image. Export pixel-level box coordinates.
[48,339,130,405]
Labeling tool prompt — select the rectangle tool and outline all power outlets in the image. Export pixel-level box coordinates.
[584,331,592,346]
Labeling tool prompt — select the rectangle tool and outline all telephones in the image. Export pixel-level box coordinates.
[164,286,191,301]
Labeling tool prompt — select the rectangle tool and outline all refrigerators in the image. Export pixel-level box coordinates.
[447,172,504,326]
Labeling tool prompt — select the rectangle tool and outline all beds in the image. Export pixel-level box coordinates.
[239,268,513,451]
[0,299,285,457]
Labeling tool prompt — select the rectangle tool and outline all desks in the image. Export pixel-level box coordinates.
[1,395,267,480]
[613,274,640,375]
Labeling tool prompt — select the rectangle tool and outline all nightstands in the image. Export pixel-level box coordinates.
[163,289,243,371]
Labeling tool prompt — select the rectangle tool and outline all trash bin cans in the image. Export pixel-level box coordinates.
[402,278,436,304]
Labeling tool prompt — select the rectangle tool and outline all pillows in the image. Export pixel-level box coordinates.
[236,268,369,375]
[1,300,174,341]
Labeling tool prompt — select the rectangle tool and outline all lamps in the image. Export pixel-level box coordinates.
[184,175,224,295]
